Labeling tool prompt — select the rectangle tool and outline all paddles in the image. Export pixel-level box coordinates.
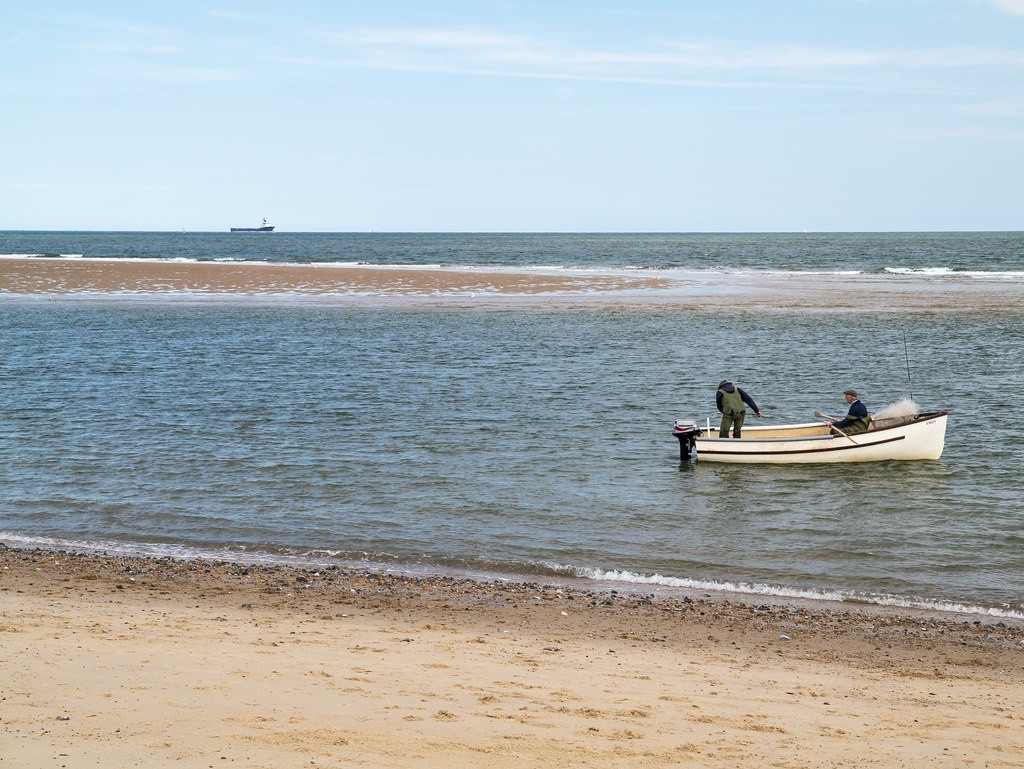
[822,420,858,444]
[814,411,841,422]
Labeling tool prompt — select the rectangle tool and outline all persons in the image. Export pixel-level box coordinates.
[716,380,761,438]
[826,390,871,438]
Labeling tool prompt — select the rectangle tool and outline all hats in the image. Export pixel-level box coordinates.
[843,390,857,397]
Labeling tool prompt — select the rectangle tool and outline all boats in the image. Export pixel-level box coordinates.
[231,226,275,231]
[672,409,948,464]
